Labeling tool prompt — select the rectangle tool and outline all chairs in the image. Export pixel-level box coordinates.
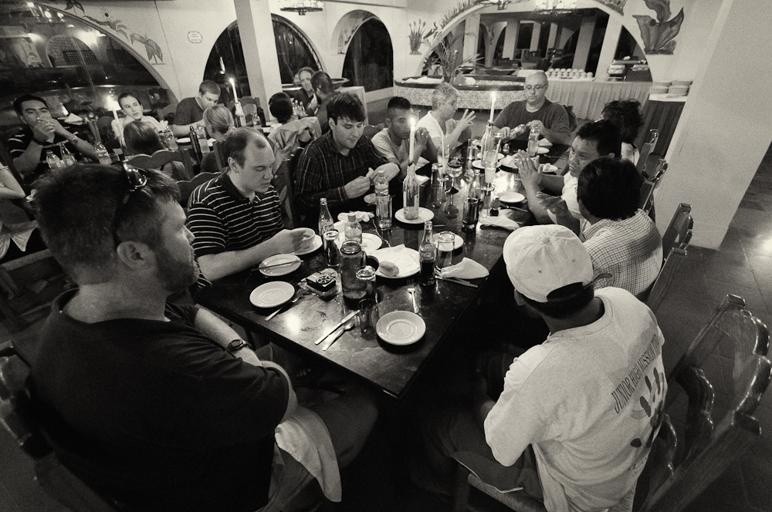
[645,202,696,313]
[449,292,767,512]
[0,339,411,512]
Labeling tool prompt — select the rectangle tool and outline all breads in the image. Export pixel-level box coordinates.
[380,261,399,277]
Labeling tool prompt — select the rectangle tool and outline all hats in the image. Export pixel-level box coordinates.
[502,223,594,303]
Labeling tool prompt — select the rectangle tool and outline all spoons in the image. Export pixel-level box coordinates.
[489,201,530,213]
[322,321,356,351]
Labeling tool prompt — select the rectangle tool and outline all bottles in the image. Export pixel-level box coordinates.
[345,214,362,247]
[60,142,76,168]
[299,102,305,118]
[468,169,481,225]
[419,221,436,288]
[196,123,210,154]
[295,100,299,110]
[46,150,65,173]
[528,124,538,156]
[164,126,178,152]
[253,113,261,130]
[375,170,388,216]
[481,120,495,166]
[292,103,296,117]
[235,102,247,127]
[319,198,334,241]
[404,160,419,221]
[95,141,112,165]
[189,126,201,155]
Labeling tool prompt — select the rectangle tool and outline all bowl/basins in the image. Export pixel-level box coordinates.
[290,227,316,249]
[650,86,670,95]
[652,78,672,87]
[667,85,690,97]
[669,80,693,87]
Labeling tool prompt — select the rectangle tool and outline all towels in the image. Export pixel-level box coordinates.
[500,154,517,169]
[440,256,490,279]
[479,212,520,232]
[338,210,374,224]
[272,405,345,505]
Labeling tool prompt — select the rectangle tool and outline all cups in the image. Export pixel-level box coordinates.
[446,161,462,196]
[430,162,444,191]
[545,68,595,80]
[468,138,479,161]
[355,267,378,309]
[434,232,456,272]
[376,196,392,230]
[322,228,342,268]
[419,243,436,287]
[462,198,483,227]
[359,298,378,339]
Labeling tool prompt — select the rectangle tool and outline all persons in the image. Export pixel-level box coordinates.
[598,100,641,168]
[111,92,174,147]
[125,122,192,182]
[414,83,476,156]
[57,88,95,114]
[6,96,101,179]
[78,100,113,139]
[513,120,623,225]
[0,162,48,263]
[201,106,237,172]
[371,96,440,176]
[31,162,382,511]
[293,68,315,113]
[491,71,570,143]
[309,72,342,134]
[186,127,306,296]
[268,91,320,228]
[294,93,402,221]
[510,157,664,349]
[172,80,221,136]
[409,225,667,512]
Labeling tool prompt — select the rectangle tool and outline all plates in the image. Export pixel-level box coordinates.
[395,206,434,225]
[526,146,549,154]
[369,246,423,279]
[472,159,501,170]
[259,253,302,277]
[249,280,296,309]
[499,156,520,168]
[476,151,506,160]
[375,309,427,347]
[288,234,322,256]
[335,232,383,256]
[536,163,559,173]
[428,230,465,252]
[496,191,526,204]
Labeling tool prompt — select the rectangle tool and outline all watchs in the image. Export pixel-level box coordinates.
[226,339,252,354]
[70,135,78,144]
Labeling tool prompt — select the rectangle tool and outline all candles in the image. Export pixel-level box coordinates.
[229,76,238,105]
[108,95,121,120]
[409,116,418,159]
[489,90,497,123]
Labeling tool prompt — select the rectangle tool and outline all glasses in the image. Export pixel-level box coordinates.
[111,156,148,256]
[523,84,549,90]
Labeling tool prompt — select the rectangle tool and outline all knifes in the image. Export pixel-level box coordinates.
[264,296,303,322]
[314,309,361,346]
[249,259,304,272]
